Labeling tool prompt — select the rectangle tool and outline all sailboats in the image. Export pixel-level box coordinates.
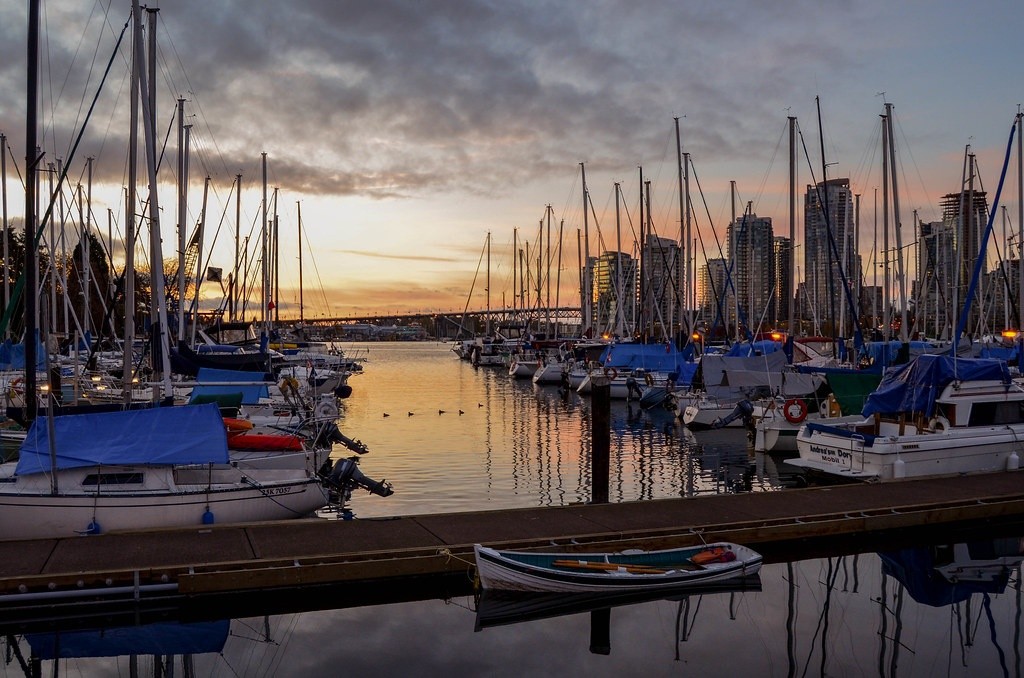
[2,618,278,678]
[451,90,1023,480]
[0,0,388,537]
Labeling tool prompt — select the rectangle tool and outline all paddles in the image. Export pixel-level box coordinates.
[552,557,697,574]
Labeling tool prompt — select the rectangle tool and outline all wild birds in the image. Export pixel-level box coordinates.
[459,410,464,413]
[408,412,414,415]
[439,410,445,413]
[383,413,390,417]
[478,403,483,406]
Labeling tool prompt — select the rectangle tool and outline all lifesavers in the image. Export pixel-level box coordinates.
[315,401,338,424]
[604,367,617,380]
[281,378,299,398]
[783,398,808,424]
[644,374,656,388]
[927,416,949,438]
[584,354,591,364]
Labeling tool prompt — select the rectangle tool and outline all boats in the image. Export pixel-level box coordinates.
[473,542,764,591]
